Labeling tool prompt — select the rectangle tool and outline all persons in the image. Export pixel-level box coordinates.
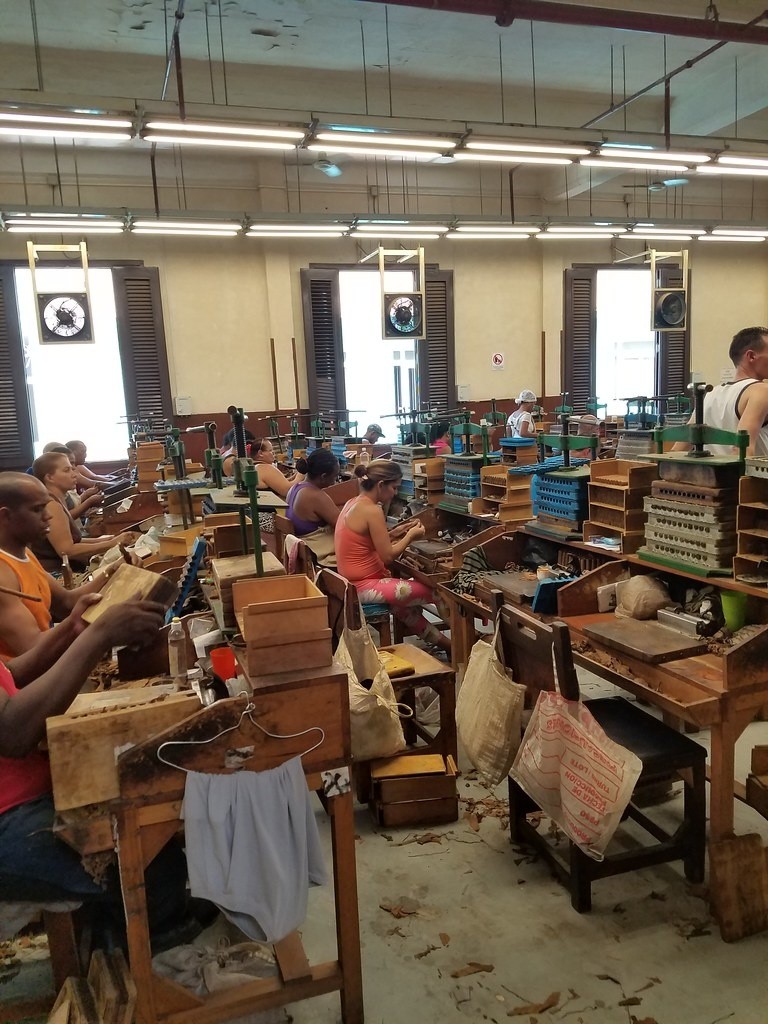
[218,427,307,534]
[670,326,768,457]
[333,460,496,663]
[505,390,539,438]
[1,592,222,959]
[570,414,604,460]
[429,421,451,454]
[361,424,386,444]
[283,447,340,570]
[22,439,137,588]
[0,470,143,660]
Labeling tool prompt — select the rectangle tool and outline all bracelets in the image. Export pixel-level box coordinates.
[100,570,110,580]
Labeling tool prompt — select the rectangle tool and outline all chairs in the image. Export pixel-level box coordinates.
[0,899,83,1024]
[271,508,709,915]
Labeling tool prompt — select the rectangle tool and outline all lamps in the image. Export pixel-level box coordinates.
[0,91,768,244]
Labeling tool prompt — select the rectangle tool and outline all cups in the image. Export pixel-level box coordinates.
[537,569,548,581]
[612,416,618,423]
[720,590,748,631]
[210,647,235,682]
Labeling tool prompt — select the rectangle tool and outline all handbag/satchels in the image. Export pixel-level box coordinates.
[455,608,528,786]
[507,641,644,862]
[331,584,413,765]
[151,935,288,1024]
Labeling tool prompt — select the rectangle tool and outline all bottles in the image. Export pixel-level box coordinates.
[168,617,187,677]
[360,448,369,467]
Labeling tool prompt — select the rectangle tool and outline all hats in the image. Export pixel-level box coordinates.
[367,424,386,438]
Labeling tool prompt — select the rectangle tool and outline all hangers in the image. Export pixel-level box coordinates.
[157,690,325,774]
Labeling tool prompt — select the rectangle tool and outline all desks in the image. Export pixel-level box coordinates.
[392,539,768,919]
[108,755,366,1024]
